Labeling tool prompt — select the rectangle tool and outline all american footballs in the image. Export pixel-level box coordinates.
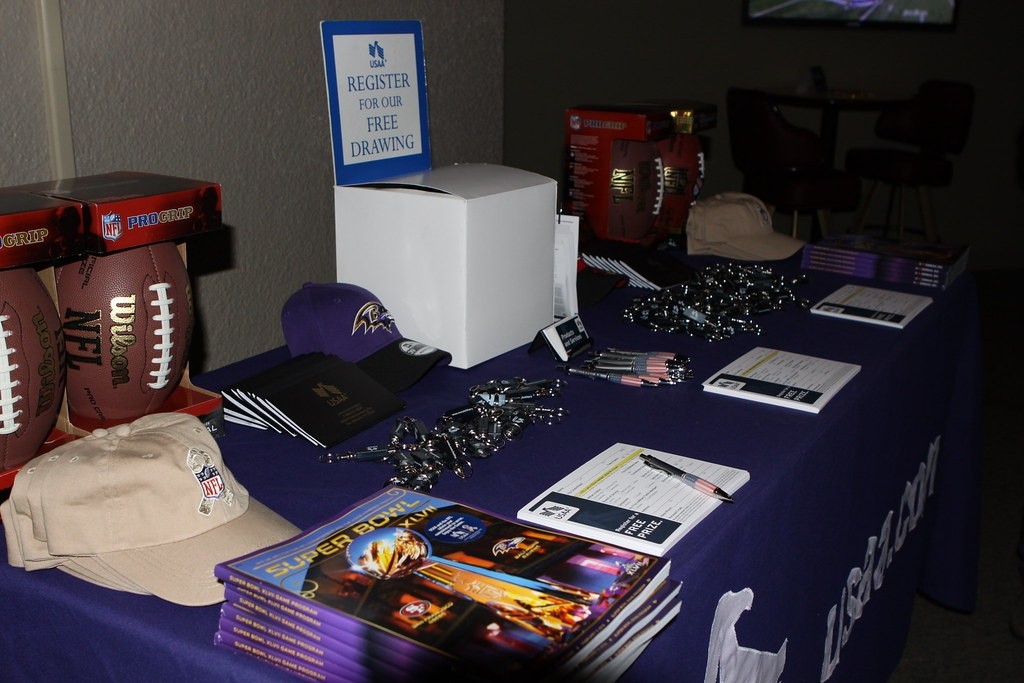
[0,264,68,471]
[656,133,707,230]
[52,236,196,432]
[609,137,665,241]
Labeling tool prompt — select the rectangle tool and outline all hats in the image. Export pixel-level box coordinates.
[0,412,305,607]
[281,280,452,393]
[686,192,807,261]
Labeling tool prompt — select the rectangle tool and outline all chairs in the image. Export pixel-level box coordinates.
[727,80,976,246]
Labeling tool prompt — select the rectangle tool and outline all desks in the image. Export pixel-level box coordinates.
[0,265,984,683]
[758,87,912,243]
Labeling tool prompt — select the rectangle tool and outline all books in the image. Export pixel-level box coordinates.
[800,234,950,290]
[215,441,751,677]
[702,347,862,414]
[221,352,408,448]
[810,284,933,329]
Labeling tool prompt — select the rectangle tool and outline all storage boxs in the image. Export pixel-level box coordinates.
[0,169,222,270]
[564,98,717,248]
[331,162,558,369]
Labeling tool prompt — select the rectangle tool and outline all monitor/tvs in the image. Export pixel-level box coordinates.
[739,0,960,31]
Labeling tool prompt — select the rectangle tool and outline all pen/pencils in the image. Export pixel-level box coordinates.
[639,452,733,505]
[558,346,693,388]
